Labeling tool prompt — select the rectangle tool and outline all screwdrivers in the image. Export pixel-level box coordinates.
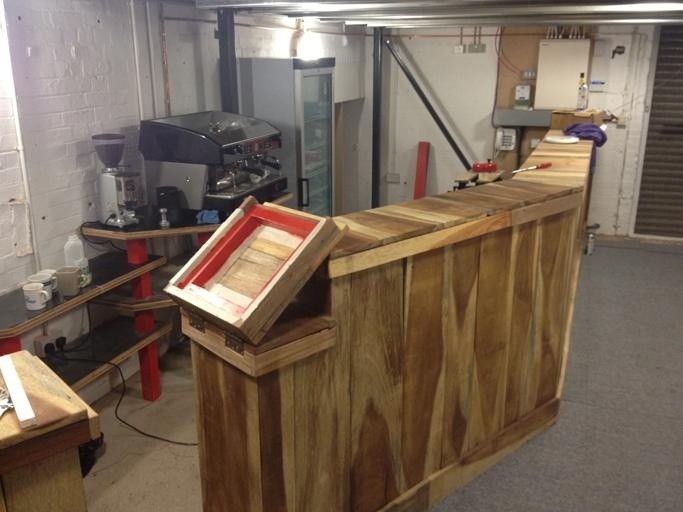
[512,162,552,173]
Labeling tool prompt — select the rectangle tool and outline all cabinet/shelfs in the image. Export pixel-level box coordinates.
[0,191,297,402]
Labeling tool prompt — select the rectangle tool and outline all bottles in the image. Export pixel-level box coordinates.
[577,72,588,110]
[587,230,595,256]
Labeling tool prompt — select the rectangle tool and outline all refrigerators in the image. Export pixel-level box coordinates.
[239,55,336,220]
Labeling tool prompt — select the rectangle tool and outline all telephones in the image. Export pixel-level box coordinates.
[494,127,516,151]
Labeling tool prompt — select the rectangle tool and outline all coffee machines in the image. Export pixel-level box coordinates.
[91,133,145,230]
[138,110,287,216]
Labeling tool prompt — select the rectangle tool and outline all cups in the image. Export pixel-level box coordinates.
[23,282,49,311]
[56,266,87,296]
[28,269,59,302]
[156,185,179,211]
